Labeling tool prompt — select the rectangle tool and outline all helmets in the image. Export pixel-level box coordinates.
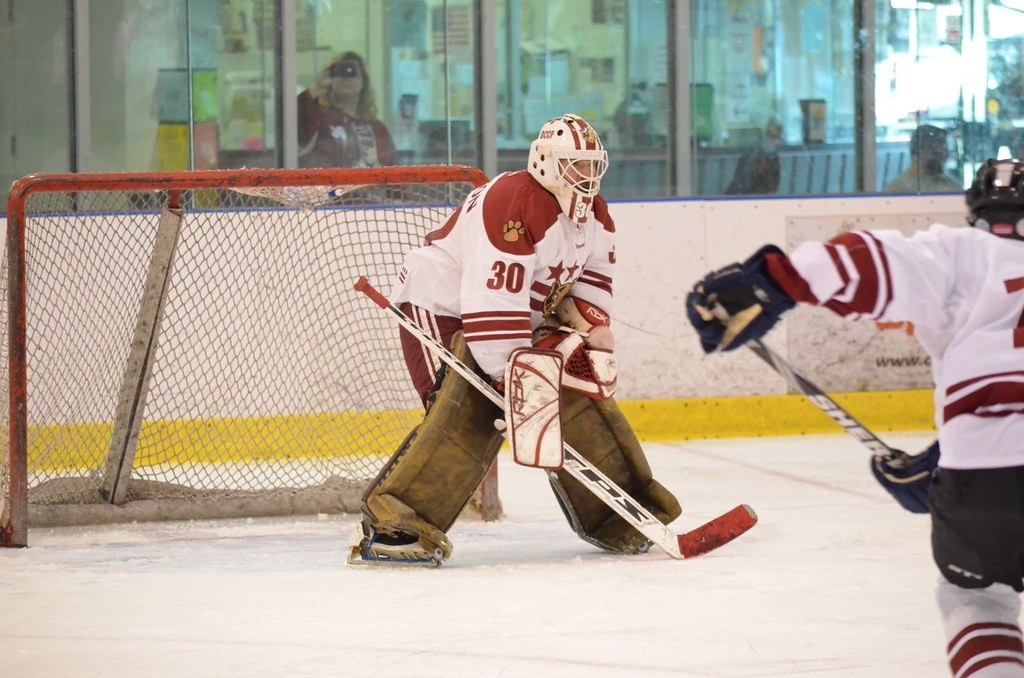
[965,158,1024,212]
[527,113,603,225]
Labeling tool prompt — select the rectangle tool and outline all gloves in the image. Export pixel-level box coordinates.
[686,244,799,354]
[872,439,941,513]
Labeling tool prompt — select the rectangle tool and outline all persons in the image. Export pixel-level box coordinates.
[883,126,963,193]
[297,51,397,168]
[724,118,787,195]
[612,81,654,195]
[361,112,682,562]
[685,158,1024,678]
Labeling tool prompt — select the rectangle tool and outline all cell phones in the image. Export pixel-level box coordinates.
[330,62,356,78]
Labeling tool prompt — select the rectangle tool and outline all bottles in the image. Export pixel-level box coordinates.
[292,187,343,201]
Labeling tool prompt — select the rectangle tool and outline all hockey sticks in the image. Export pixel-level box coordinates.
[743,336,899,460]
[350,273,761,562]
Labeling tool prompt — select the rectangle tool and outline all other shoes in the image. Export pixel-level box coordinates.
[360,520,433,561]
